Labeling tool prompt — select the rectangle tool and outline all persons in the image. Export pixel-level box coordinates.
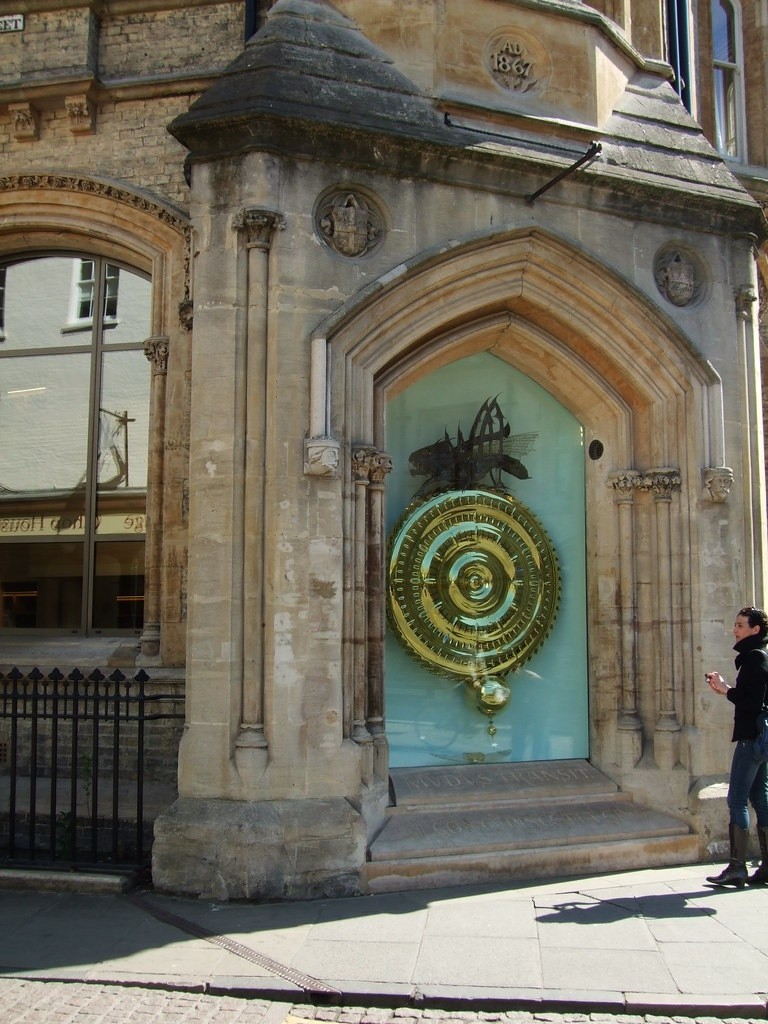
[703,607,768,888]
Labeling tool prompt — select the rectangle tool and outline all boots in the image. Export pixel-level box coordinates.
[707,822,751,890]
[747,824,768,884]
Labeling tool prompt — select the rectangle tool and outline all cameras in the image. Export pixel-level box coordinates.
[704,674,712,683]
